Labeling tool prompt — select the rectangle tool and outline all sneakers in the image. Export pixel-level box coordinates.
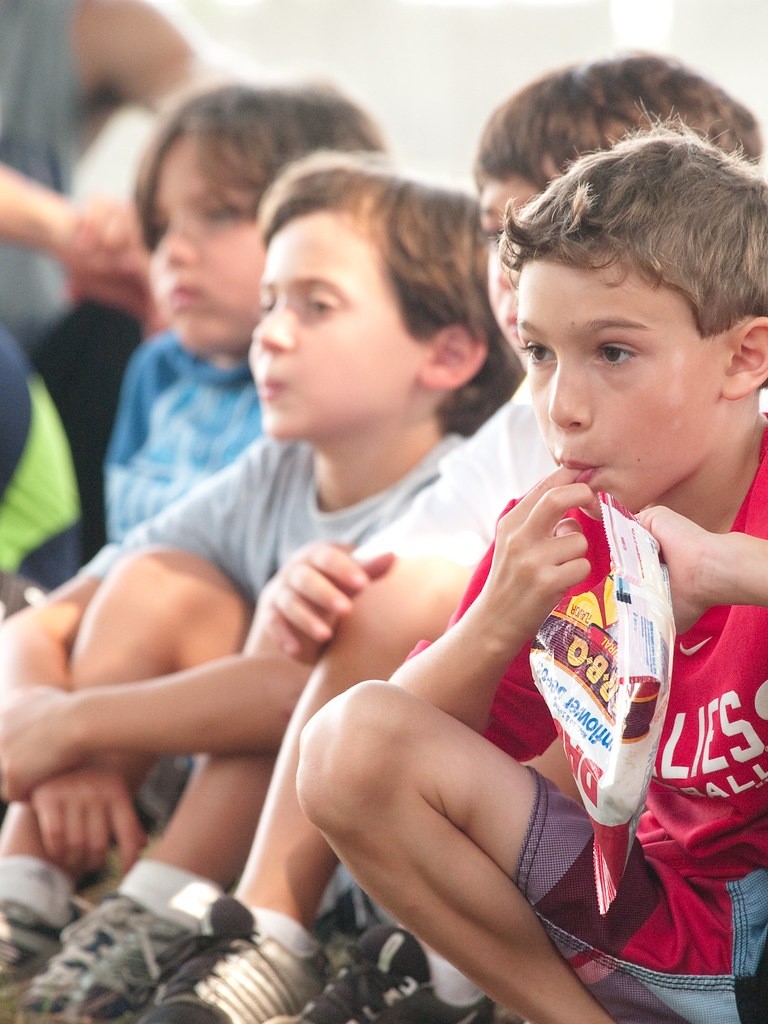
[13,887,203,1023]
[260,923,496,1024]
[1,854,93,996]
[130,897,325,1023]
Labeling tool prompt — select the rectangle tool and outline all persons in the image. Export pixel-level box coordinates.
[297,94,768,1024]
[101,84,389,825]
[140,51,765,1022]
[0,0,249,578]
[2,149,530,1024]
